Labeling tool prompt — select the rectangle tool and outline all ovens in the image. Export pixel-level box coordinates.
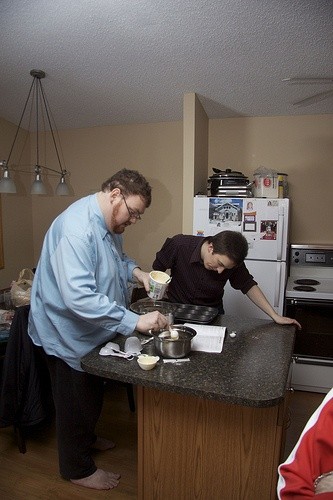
[283,298,333,365]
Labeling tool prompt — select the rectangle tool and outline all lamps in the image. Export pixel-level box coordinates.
[0,68,75,197]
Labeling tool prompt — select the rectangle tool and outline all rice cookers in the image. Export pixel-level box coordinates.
[253,173,279,199]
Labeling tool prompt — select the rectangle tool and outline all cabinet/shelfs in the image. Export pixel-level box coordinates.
[138,353,298,500]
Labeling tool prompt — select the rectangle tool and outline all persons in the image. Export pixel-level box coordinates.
[28,169,170,490]
[277,387,333,500]
[152,231,302,329]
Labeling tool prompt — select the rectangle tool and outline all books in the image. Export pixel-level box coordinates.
[183,323,226,353]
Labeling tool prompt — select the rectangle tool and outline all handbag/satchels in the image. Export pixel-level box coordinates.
[10,268,35,307]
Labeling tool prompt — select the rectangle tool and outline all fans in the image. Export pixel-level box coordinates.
[281,77,333,108]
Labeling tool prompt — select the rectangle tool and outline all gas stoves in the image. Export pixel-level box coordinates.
[284,243,333,299]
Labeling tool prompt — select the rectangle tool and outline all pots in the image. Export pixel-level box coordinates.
[150,325,196,358]
[207,168,254,196]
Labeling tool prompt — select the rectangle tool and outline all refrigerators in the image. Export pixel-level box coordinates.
[193,196,289,320]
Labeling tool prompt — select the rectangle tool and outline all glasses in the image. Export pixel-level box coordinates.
[120,192,141,220]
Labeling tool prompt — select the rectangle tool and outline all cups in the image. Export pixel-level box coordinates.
[137,355,159,371]
[277,173,287,198]
[147,271,171,300]
[125,337,144,353]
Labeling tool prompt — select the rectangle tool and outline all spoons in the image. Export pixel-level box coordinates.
[212,167,242,174]
[170,326,179,340]
